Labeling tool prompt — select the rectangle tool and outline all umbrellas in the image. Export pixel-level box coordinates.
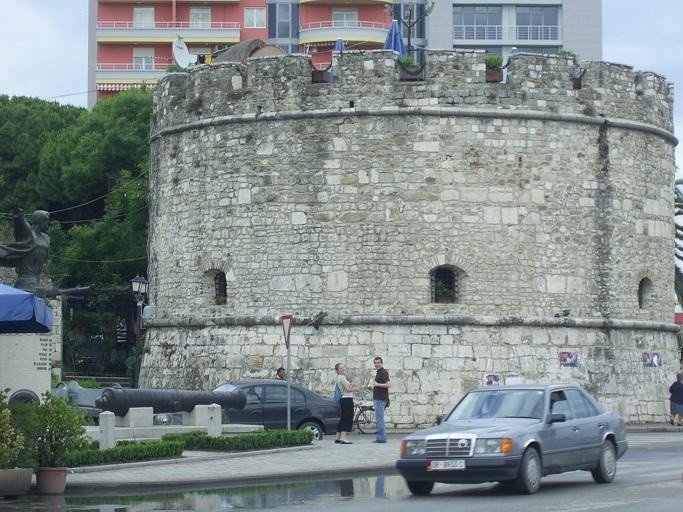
[382,19,408,65]
[329,36,347,83]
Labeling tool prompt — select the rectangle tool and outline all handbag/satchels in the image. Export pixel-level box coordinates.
[385,398,391,408]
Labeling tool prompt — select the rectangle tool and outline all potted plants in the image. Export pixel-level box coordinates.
[401,55,423,80]
[485,54,503,83]
[557,50,581,90]
[0,386,91,494]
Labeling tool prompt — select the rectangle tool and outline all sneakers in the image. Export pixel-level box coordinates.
[372,439,387,443]
[670,419,682,425]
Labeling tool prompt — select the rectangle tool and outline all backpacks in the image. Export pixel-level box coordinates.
[333,381,346,404]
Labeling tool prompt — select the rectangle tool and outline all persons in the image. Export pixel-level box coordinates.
[338,476,354,502]
[641,352,660,366]
[669,373,683,427]
[1,204,101,303]
[371,473,388,499]
[66,312,137,375]
[276,367,287,380]
[367,357,391,443]
[487,374,500,386]
[560,352,576,366]
[332,362,356,445]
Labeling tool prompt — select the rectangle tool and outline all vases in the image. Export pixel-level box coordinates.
[311,70,330,83]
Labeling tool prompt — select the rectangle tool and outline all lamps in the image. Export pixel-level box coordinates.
[132,273,148,330]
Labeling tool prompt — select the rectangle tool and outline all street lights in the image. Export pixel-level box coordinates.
[130,273,148,387]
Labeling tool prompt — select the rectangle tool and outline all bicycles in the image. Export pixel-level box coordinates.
[353,402,378,435]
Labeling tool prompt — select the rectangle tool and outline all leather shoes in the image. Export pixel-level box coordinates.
[334,440,354,444]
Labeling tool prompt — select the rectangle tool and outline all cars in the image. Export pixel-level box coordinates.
[398,383,628,495]
[154,378,341,440]
